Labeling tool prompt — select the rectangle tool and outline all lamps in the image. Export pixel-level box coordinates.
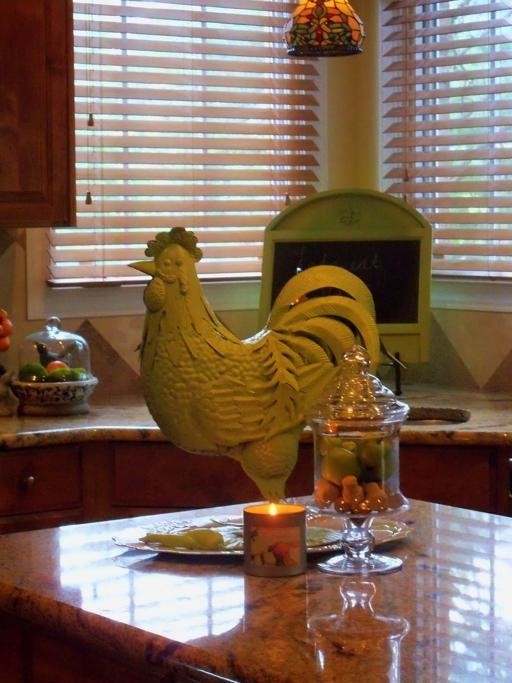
[283,0,367,57]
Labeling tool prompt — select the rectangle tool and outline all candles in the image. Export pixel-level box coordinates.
[242,503,307,577]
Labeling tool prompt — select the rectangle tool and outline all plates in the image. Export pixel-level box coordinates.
[111,513,407,557]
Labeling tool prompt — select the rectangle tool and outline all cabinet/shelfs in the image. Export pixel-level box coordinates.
[0,446,512,532]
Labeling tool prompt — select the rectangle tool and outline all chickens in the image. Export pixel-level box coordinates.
[128,228,381,502]
